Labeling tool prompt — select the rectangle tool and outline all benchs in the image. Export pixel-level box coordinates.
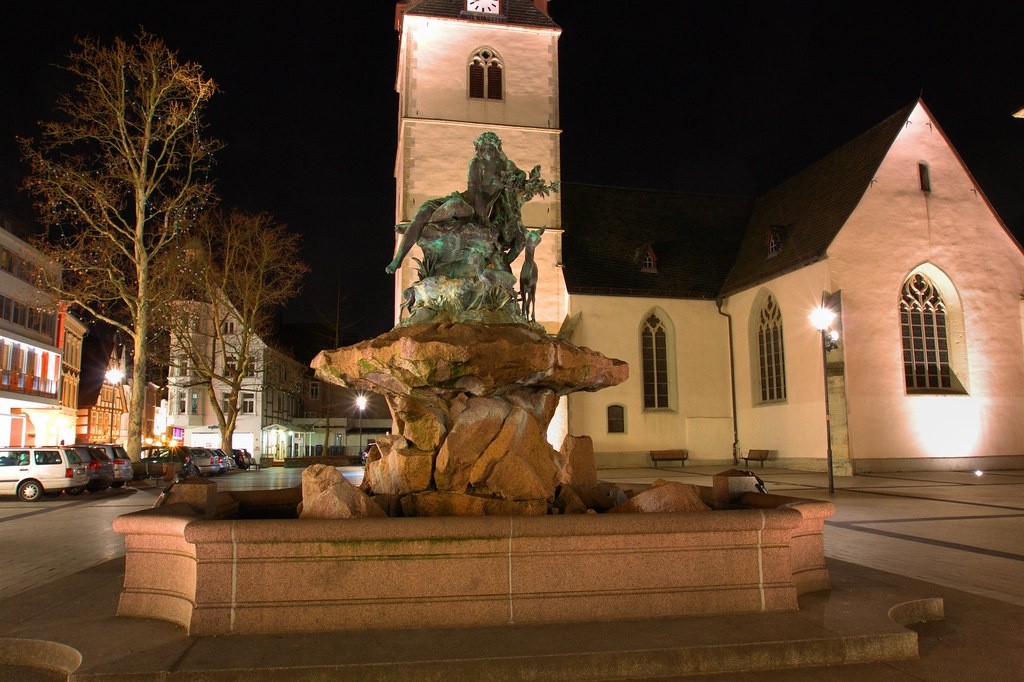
[249,458,263,472]
[650,450,688,467]
[243,458,258,472]
[739,449,770,468]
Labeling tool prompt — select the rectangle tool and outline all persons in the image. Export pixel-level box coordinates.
[184,457,200,479]
[386,132,527,273]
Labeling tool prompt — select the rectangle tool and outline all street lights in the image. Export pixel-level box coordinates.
[813,306,842,492]
[356,396,366,464]
[105,368,122,443]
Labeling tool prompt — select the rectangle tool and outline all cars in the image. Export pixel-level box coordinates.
[136,443,252,482]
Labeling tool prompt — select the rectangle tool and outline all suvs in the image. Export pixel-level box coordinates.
[86,442,134,489]
[0,444,90,502]
[63,441,116,493]
